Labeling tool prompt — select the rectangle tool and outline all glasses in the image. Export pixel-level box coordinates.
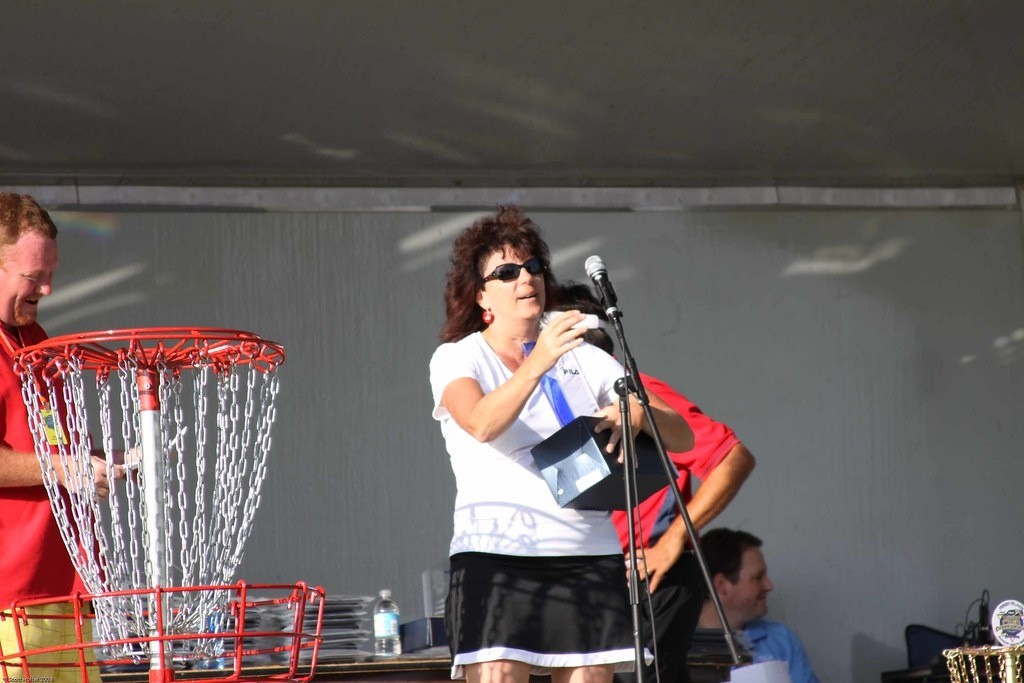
[483,258,548,284]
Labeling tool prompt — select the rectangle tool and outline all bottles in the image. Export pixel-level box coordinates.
[198,609,225,670]
[374,590,401,655]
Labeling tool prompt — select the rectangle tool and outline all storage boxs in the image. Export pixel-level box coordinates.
[530,415,681,511]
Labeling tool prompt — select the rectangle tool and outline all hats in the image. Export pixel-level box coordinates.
[536,308,610,333]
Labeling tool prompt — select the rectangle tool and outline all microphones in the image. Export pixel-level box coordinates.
[583,253,626,342]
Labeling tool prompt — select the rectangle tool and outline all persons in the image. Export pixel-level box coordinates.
[0,193,175,683]
[427,213,697,683]
[682,528,820,683]
[544,284,759,683]
